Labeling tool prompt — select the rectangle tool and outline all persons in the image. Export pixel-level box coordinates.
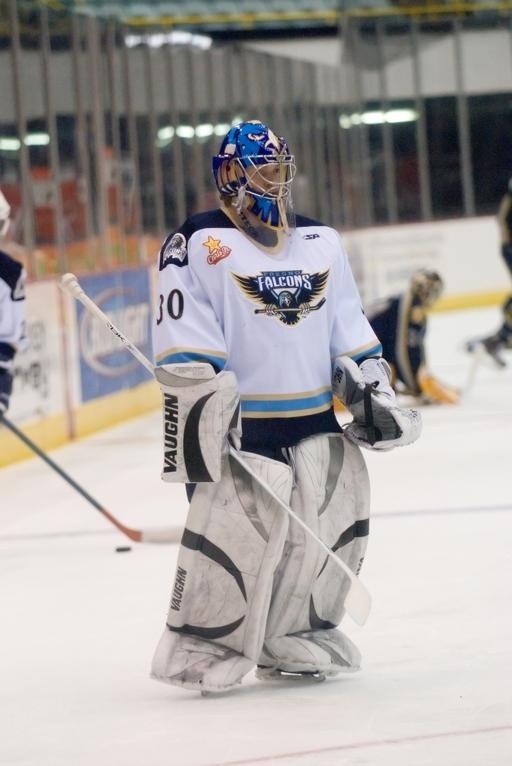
[467,176,511,367]
[362,267,447,397]
[1,193,29,429]
[138,116,424,698]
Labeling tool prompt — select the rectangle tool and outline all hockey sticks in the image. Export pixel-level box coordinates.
[56,273,372,627]
[0,413,186,544]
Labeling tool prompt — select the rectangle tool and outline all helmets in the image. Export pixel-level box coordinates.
[412,269,443,306]
[213,119,295,230]
